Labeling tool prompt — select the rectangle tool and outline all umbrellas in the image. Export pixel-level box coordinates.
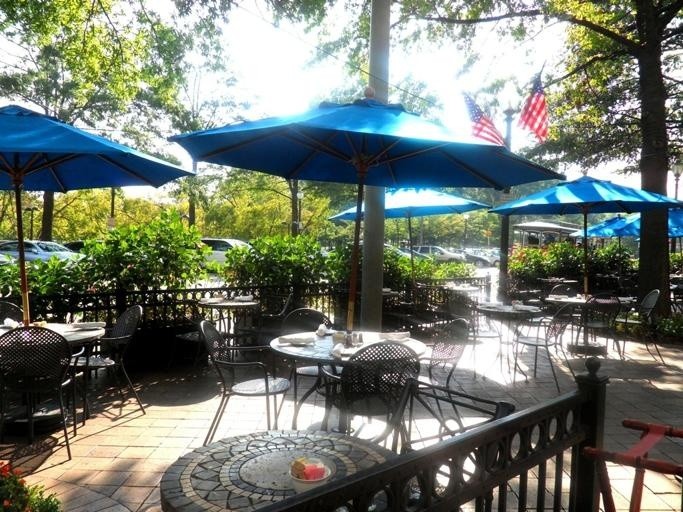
[565,207,626,248]
[0,104,193,327]
[484,173,683,343]
[166,87,568,348]
[329,183,492,287]
[597,206,682,237]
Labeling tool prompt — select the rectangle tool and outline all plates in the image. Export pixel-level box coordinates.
[288,464,332,483]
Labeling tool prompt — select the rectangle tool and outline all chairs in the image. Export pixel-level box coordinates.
[0,299,146,459]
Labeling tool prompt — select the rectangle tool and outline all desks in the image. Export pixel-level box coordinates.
[157,427,398,511]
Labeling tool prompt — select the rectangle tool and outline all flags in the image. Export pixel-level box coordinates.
[459,89,507,147]
[521,64,551,142]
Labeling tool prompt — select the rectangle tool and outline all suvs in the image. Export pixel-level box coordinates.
[412,246,466,264]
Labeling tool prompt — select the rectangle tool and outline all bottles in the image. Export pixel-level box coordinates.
[344,329,363,345]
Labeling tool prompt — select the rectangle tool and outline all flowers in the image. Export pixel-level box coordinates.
[0,460,61,512]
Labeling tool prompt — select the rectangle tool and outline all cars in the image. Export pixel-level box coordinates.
[198,238,252,271]
[320,242,412,258]
[453,249,490,268]
[0,241,83,268]
[462,248,499,267]
[244,240,252,246]
[487,249,500,256]
[64,240,103,253]
[0,250,14,269]
[399,248,429,260]
